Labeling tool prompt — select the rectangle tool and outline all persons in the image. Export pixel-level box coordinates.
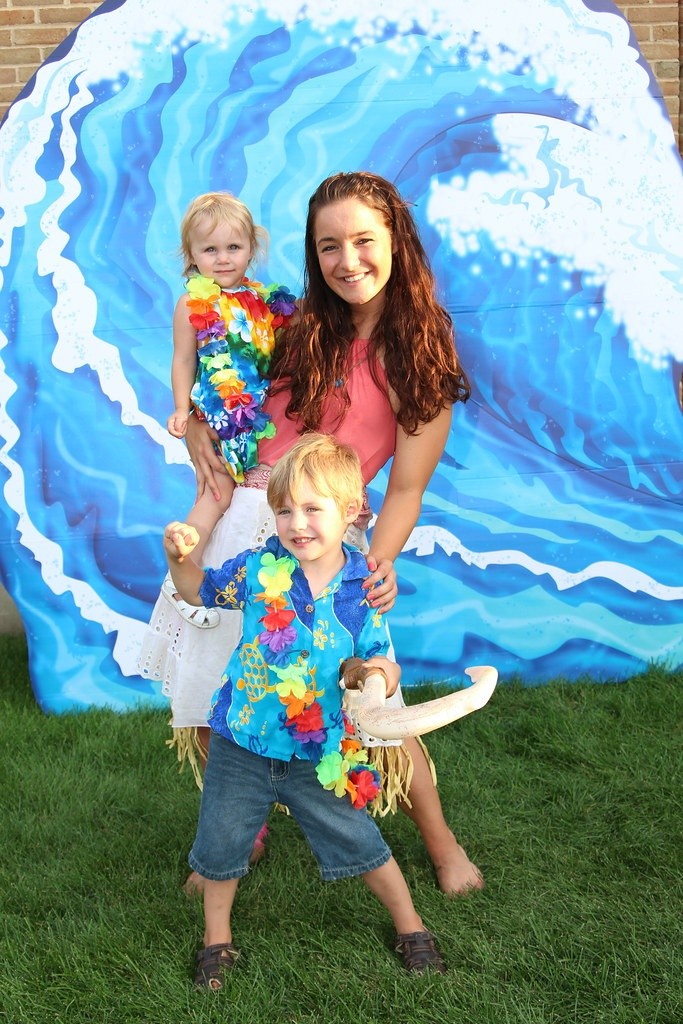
[163,432,448,991]
[161,191,296,628]
[137,170,485,897]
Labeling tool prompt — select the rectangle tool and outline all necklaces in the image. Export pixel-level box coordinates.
[334,339,385,387]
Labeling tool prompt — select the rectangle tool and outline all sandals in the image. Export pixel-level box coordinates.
[395,925,446,977]
[194,938,234,992]
[161,568,220,628]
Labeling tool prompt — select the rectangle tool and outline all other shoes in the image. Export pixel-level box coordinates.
[184,842,263,895]
[436,845,484,896]
